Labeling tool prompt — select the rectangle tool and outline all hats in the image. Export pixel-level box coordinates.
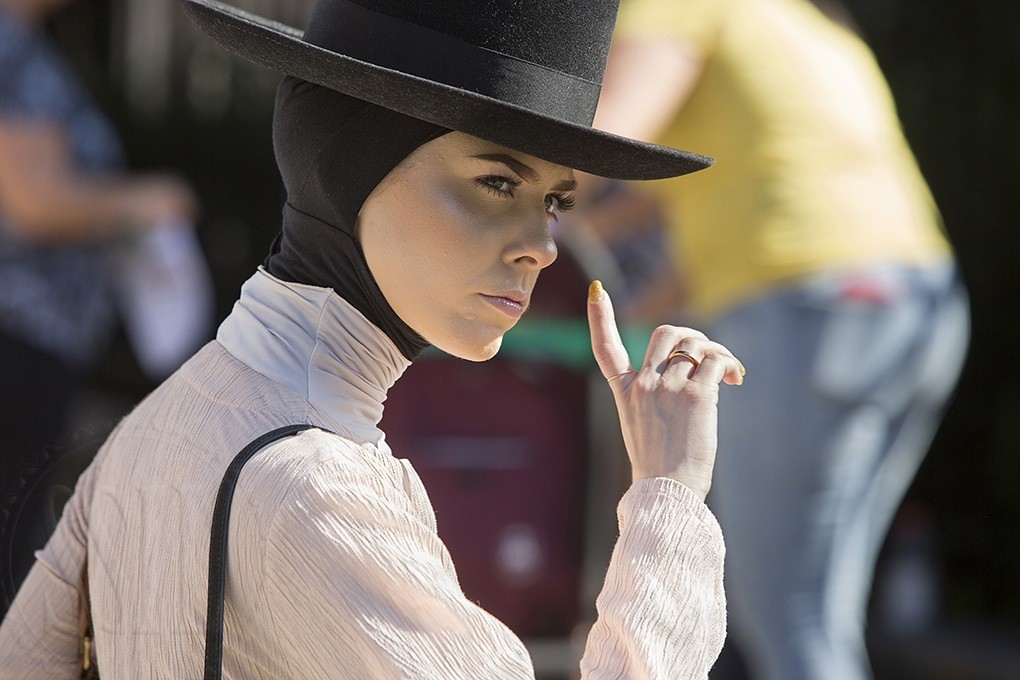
[183,2,712,182]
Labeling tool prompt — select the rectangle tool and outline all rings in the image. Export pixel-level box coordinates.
[668,349,700,366]
[607,369,634,382]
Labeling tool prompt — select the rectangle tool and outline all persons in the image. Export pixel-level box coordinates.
[2,0,749,680]
[563,0,969,680]
[0,0,214,625]
